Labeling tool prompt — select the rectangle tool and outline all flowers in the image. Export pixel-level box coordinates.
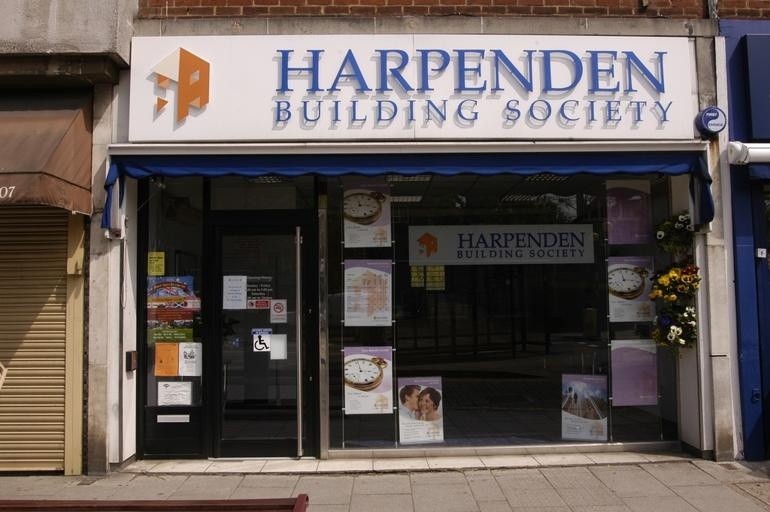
[648,208,702,349]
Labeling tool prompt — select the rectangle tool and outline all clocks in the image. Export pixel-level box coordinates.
[343,357,388,392]
[343,191,386,226]
[608,266,649,300]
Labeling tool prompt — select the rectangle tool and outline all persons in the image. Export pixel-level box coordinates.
[399,385,421,420]
[414,387,441,421]
